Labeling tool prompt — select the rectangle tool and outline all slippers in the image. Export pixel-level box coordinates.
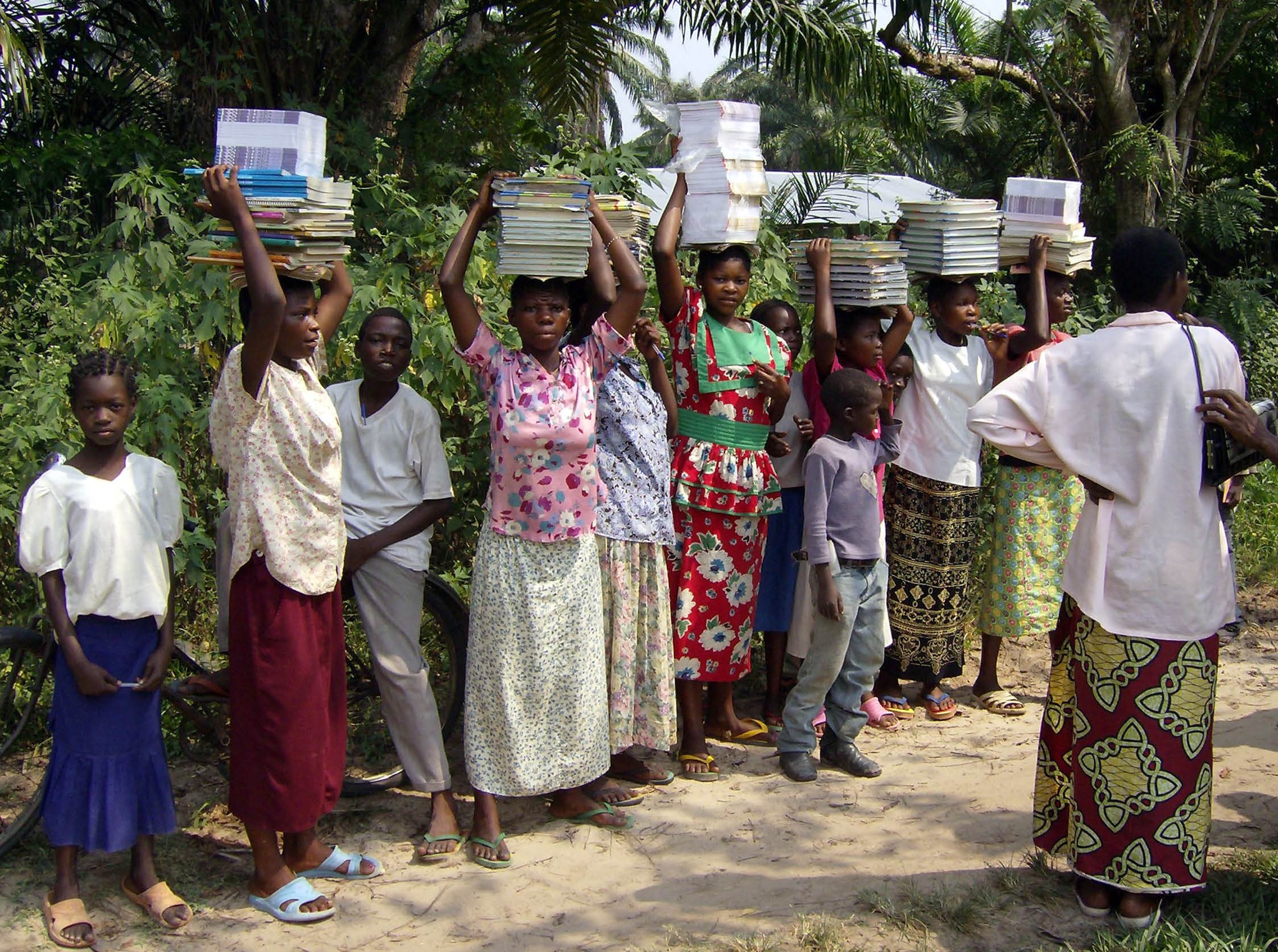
[248,875,335,921]
[1119,906,1158,929]
[556,802,635,833]
[420,826,469,860]
[40,890,97,947]
[763,709,784,732]
[470,832,511,869]
[811,705,826,736]
[713,718,780,748]
[677,753,720,782]
[918,690,957,720]
[971,690,1026,715]
[605,760,674,786]
[297,843,381,881]
[121,874,194,928]
[878,691,913,719]
[1071,874,1111,917]
[862,696,900,731]
[585,781,644,806]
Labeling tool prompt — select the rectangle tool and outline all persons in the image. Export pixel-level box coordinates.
[18,139,1278,949]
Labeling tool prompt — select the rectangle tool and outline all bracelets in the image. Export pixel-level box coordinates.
[604,237,621,254]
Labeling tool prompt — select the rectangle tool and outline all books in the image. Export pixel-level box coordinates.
[180,164,358,283]
[488,100,1095,307]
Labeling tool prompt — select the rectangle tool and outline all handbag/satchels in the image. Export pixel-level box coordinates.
[1181,319,1278,486]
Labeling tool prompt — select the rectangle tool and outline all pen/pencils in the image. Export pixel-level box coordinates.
[362,403,367,425]
[969,323,1007,337]
[651,342,666,360]
[109,683,141,687]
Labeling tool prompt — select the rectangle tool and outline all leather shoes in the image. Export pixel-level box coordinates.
[779,750,817,783]
[820,737,882,776]
